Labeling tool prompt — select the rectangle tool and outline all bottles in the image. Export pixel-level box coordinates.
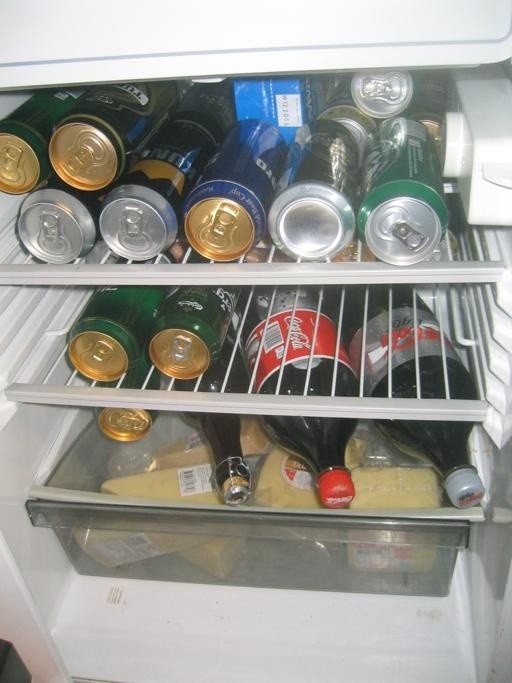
[173,320,254,505]
[229,286,362,509]
[325,285,487,509]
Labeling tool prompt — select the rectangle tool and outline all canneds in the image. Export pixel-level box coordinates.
[184,119,287,263]
[357,116,449,267]
[173,80,236,141]
[95,404,154,445]
[1,90,85,195]
[314,79,376,134]
[268,120,360,264]
[49,80,190,192]
[146,286,249,382]
[66,286,166,383]
[97,119,217,263]
[350,68,415,119]
[411,73,446,146]
[14,178,103,264]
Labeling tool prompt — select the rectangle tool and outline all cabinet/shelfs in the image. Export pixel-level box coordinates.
[0,1,512,683]
